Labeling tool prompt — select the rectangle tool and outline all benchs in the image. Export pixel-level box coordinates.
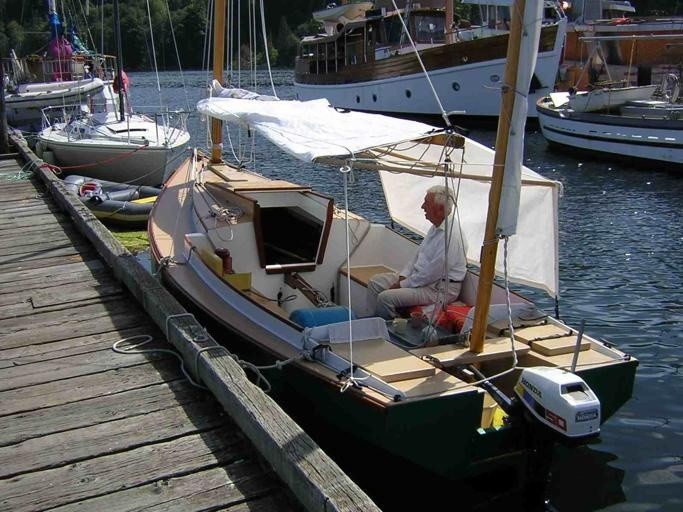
[341,266,470,334]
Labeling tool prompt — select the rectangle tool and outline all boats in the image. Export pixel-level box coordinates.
[294,0,570,126]
[536,33,683,165]
[65,175,163,222]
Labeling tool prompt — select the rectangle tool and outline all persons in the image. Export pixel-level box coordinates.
[365,185,469,321]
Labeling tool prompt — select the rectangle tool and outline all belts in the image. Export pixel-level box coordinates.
[439,279,464,283]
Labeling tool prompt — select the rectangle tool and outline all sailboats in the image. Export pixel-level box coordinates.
[1,0,118,132]
[146,0,639,449]
[37,0,193,189]
